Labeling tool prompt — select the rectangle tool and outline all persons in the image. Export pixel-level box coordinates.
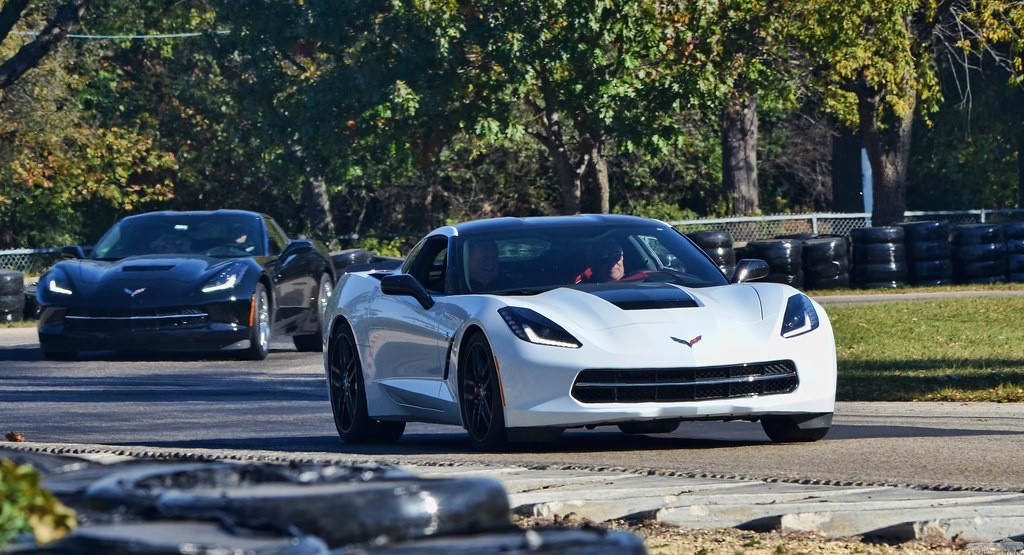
[216,228,257,252]
[459,237,533,292]
[569,237,647,287]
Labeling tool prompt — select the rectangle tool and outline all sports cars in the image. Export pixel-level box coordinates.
[320,214,837,457]
[36,209,333,358]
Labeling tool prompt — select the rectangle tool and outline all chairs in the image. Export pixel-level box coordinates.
[149,220,588,292]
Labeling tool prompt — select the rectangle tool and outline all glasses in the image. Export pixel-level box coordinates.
[595,251,624,264]
